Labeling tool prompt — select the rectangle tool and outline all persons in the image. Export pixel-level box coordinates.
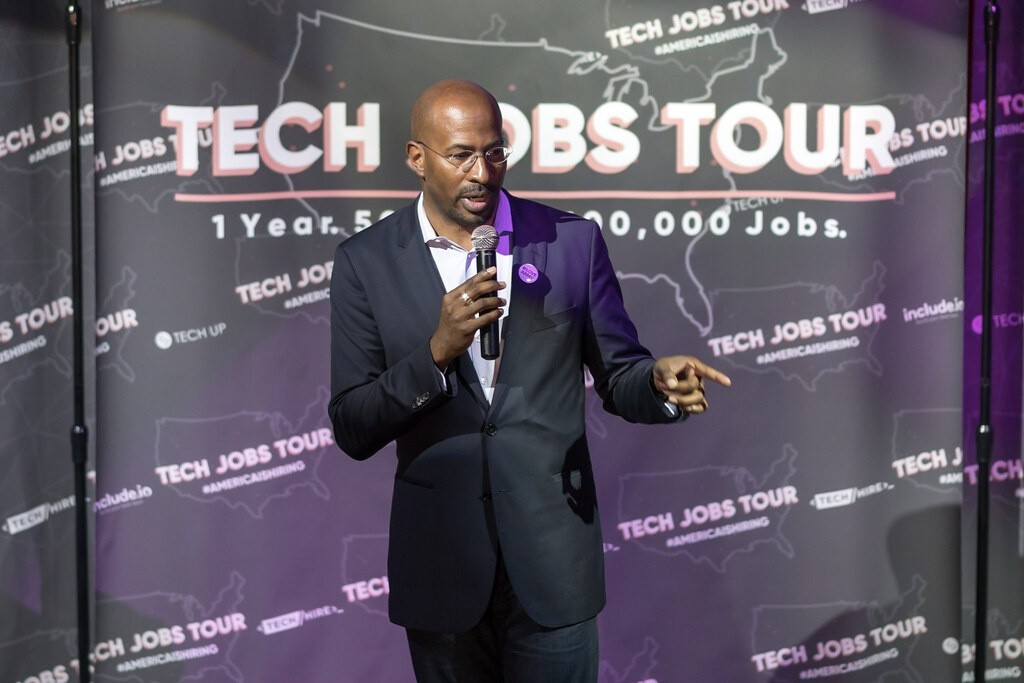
[328,79,731,683]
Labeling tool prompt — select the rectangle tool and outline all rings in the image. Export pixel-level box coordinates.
[462,293,473,305]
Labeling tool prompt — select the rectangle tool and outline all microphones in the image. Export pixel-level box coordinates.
[471,225,501,360]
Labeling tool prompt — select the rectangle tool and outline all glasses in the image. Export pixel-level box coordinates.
[416,138,513,169]
